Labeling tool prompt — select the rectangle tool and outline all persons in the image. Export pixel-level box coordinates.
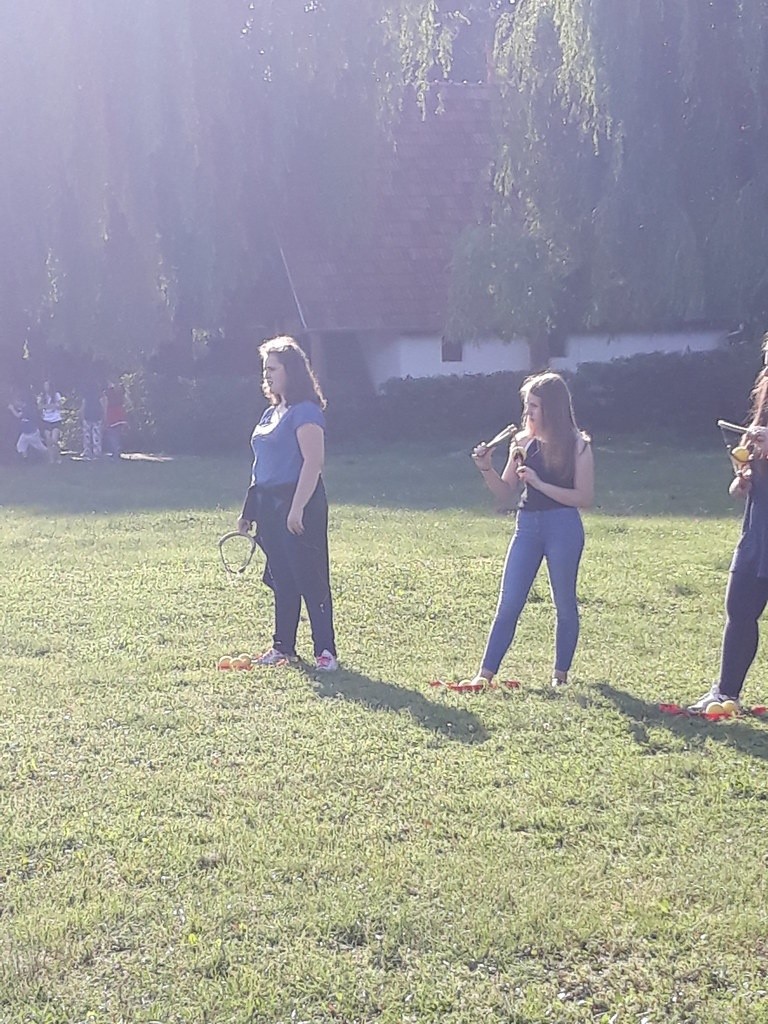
[689,333,768,711]
[238,335,338,674]
[473,374,595,685]
[7,378,131,462]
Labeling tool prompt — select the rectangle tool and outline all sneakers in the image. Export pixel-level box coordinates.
[687,684,739,713]
[254,649,296,664]
[315,649,337,672]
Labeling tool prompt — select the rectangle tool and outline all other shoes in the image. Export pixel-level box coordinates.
[551,677,567,688]
[471,677,490,686]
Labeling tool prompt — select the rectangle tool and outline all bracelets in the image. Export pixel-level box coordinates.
[479,469,493,475]
